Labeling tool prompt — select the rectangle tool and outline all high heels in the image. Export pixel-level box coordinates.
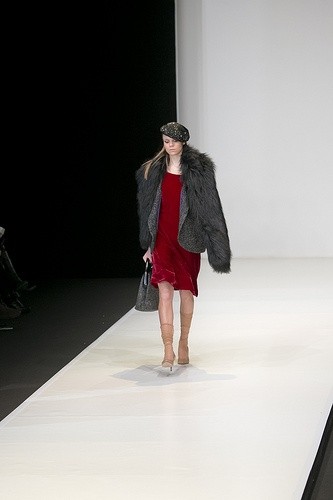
[178,347,189,364]
[161,352,175,371]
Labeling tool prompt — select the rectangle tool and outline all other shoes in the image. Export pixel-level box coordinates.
[0,283,38,319]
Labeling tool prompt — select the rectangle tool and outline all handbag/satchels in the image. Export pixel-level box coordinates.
[135,259,159,312]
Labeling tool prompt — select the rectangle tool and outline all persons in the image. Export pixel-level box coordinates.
[135,121,233,367]
[0,225,38,320]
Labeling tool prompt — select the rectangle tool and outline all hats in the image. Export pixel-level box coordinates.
[160,122,189,142]
